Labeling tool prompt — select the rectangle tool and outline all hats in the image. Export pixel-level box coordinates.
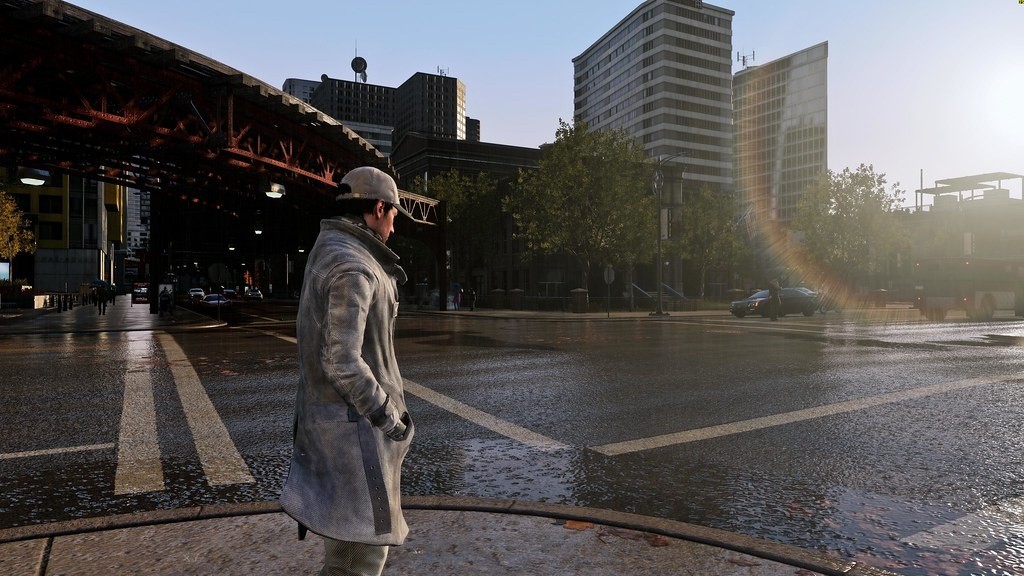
[336,166,414,221]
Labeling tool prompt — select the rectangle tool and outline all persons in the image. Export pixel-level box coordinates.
[768,275,781,321]
[453,288,464,310]
[277,165,416,575]
[92,284,116,315]
[467,288,476,311]
[159,287,169,297]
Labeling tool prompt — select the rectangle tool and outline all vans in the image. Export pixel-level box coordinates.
[914,255,1024,321]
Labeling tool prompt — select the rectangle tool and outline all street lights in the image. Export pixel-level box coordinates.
[648,150,691,317]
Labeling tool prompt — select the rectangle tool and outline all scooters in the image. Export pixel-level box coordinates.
[158,293,174,318]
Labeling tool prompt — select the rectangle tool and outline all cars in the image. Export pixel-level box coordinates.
[729,287,819,318]
[131,284,150,304]
[244,289,263,301]
[188,288,242,314]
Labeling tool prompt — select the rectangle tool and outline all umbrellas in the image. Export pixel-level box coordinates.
[92,280,118,288]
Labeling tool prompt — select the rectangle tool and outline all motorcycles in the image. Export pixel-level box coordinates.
[819,294,845,313]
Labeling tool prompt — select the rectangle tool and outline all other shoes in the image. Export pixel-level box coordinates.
[772,317,777,321]
